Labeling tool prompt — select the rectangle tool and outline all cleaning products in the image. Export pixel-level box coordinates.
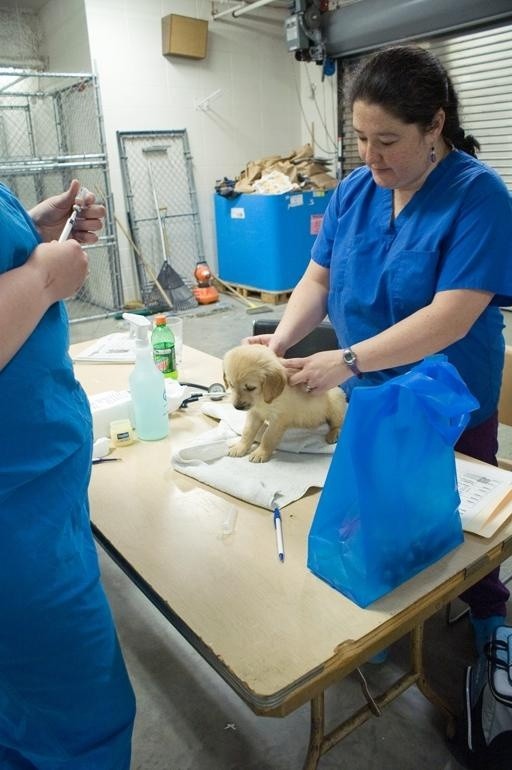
[121,312,171,440]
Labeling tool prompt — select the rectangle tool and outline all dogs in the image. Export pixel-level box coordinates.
[222,344,346,463]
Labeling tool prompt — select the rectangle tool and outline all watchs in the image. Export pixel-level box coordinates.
[343,347,361,376]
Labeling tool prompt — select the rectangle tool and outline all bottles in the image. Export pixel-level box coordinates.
[110,419,134,447]
[151,316,178,381]
[73,187,88,213]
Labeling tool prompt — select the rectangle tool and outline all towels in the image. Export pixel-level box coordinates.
[170,401,340,513]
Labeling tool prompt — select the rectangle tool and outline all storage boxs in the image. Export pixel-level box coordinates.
[212,188,337,304]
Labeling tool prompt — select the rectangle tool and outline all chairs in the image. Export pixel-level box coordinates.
[443,337,512,626]
[251,320,340,360]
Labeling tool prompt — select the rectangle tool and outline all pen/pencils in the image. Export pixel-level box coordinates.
[274,503,284,562]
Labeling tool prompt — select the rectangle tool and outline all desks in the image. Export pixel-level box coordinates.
[56,320,512,770]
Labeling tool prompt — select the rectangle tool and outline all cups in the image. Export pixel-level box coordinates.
[166,316,183,363]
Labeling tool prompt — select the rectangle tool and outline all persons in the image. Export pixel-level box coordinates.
[0,180,138,770]
[241,45,512,664]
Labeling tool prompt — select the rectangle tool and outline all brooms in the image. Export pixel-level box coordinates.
[142,160,198,312]
[212,273,274,316]
